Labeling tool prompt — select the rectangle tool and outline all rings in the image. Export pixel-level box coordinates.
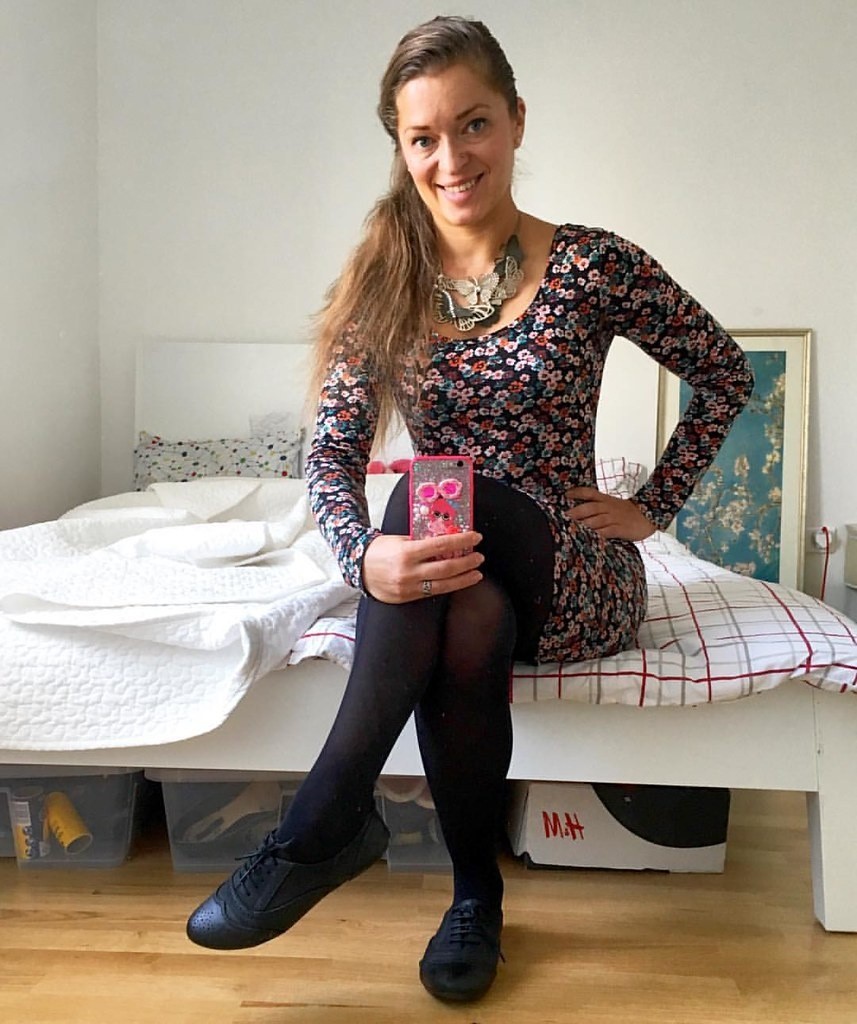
[423,581,431,595]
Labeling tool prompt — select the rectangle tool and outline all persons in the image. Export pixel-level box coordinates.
[187,16,754,1005]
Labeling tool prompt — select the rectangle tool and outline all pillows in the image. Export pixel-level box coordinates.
[132,424,307,491]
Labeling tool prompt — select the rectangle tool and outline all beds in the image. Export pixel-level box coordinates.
[0,339,857,934]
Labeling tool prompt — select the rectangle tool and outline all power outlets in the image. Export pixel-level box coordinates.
[805,527,837,554]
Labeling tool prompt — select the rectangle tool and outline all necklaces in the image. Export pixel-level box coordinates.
[428,210,524,333]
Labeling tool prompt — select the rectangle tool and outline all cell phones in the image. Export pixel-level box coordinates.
[408,456,473,562]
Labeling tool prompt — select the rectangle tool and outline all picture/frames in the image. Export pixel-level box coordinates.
[655,327,813,591]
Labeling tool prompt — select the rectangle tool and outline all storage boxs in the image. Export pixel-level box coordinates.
[144,768,309,874]
[372,775,453,874]
[0,764,144,871]
[506,780,731,875]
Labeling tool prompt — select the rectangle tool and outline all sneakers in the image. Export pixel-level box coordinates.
[187,803,391,951]
[419,900,506,1005]
